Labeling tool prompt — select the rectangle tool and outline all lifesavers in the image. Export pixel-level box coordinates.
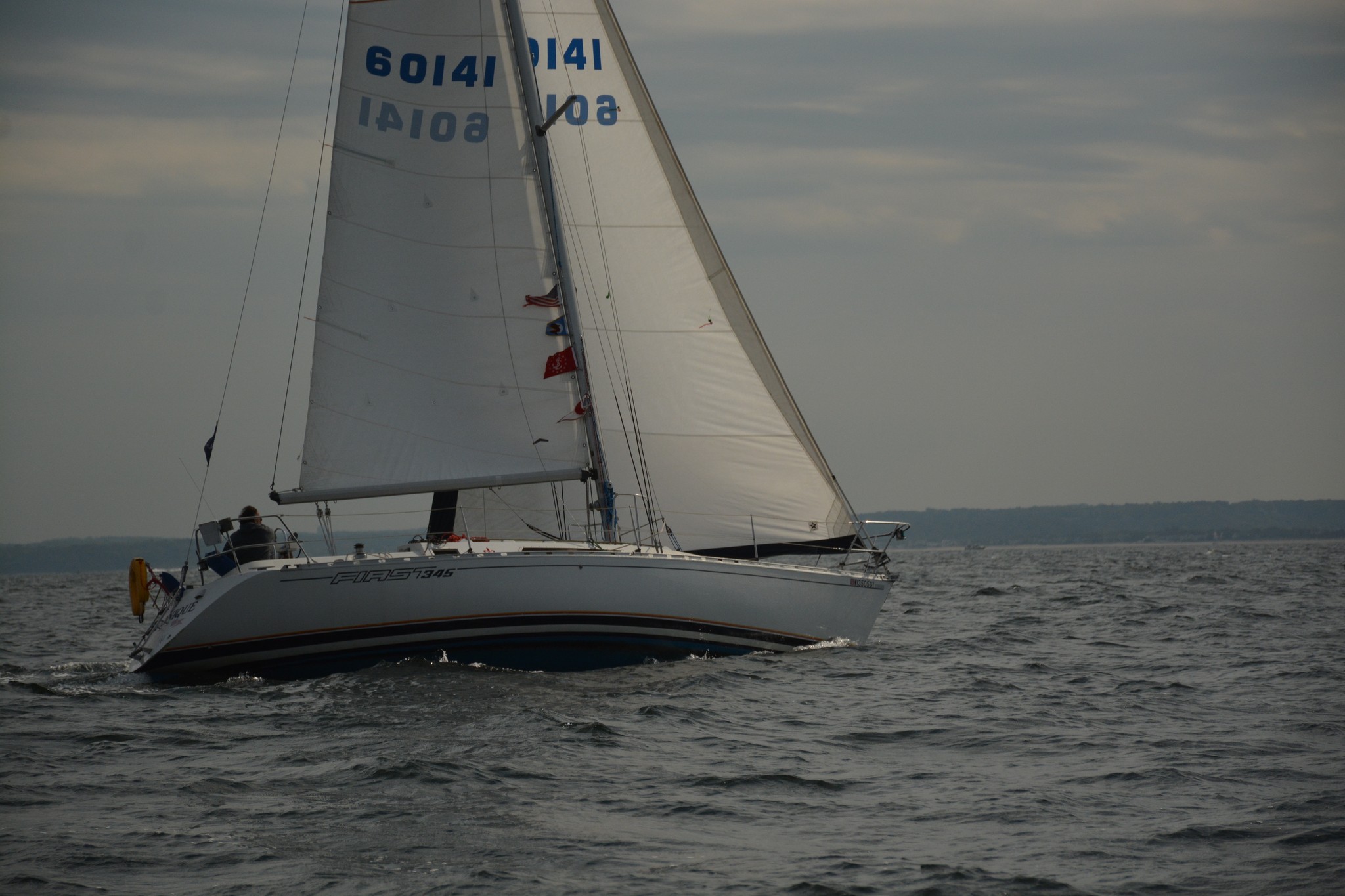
[128,557,149,617]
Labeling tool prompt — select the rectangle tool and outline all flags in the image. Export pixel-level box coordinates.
[543,345,577,380]
[522,283,561,307]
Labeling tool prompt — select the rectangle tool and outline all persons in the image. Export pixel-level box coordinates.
[221,505,280,567]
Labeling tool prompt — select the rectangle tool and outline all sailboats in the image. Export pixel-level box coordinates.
[124,1,914,689]
